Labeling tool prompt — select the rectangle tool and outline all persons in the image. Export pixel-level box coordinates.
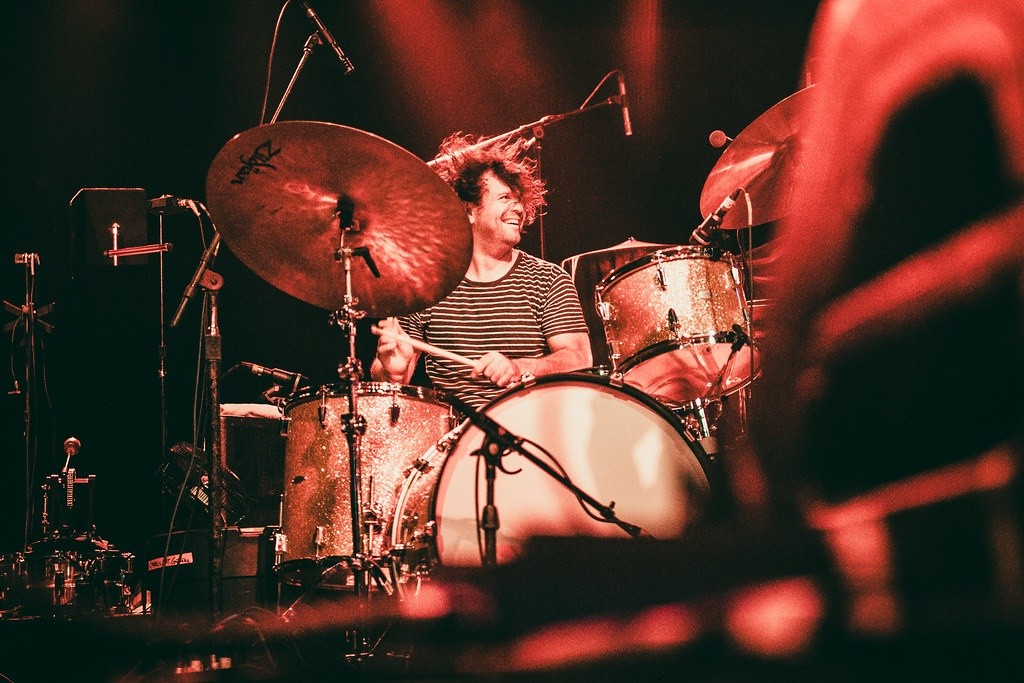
[370,132,593,425]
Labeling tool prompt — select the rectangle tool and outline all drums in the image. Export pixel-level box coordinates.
[427,372,719,567]
[594,243,765,411]
[273,381,459,594]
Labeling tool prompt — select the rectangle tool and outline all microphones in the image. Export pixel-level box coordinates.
[618,74,633,136]
[601,508,655,541]
[710,130,733,149]
[241,362,310,390]
[689,188,740,246]
[298,0,354,75]
[148,195,200,211]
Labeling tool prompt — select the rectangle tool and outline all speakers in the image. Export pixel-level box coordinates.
[143,525,276,627]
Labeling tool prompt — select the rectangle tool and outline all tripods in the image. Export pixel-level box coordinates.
[281,222,396,667]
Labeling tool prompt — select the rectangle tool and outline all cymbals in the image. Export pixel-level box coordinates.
[700,81,817,231]
[560,239,703,280]
[205,120,474,319]
